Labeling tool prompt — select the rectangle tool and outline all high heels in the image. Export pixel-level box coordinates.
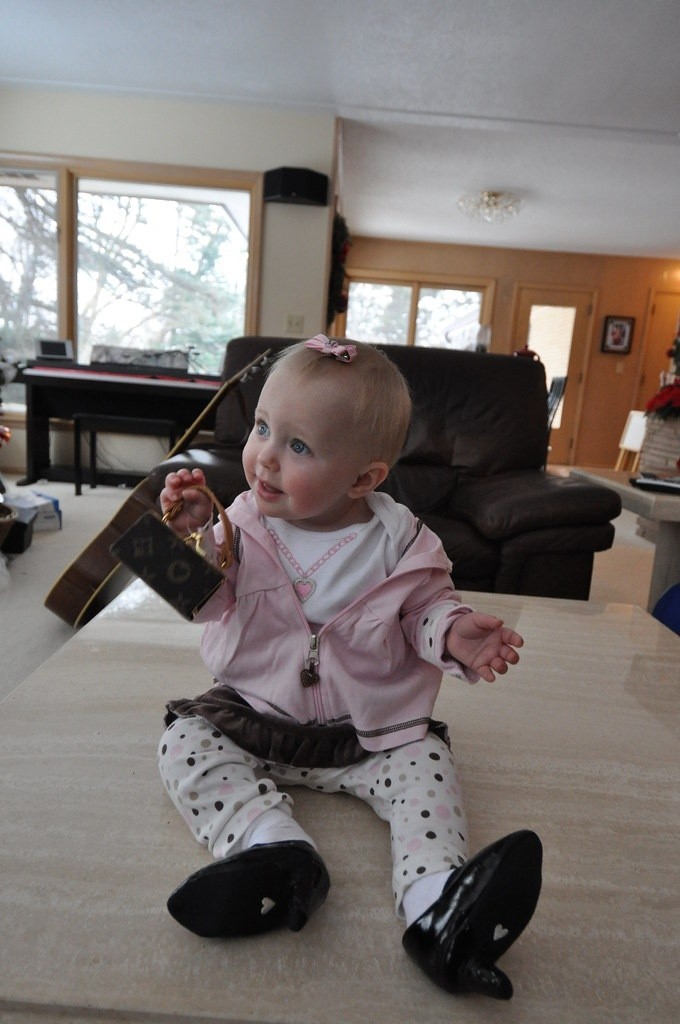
[403,829,544,999]
[168,839,331,939]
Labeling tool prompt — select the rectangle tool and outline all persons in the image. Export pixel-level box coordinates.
[153,332,543,1003]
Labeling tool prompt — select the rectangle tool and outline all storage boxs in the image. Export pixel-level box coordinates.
[24,492,62,533]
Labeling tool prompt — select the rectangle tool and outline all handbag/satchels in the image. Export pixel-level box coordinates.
[108,486,233,622]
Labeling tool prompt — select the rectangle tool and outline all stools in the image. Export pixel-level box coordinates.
[71,412,175,496]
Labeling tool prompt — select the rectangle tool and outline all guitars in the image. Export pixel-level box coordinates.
[45,346,272,627]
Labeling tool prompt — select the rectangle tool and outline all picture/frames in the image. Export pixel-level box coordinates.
[600,316,636,355]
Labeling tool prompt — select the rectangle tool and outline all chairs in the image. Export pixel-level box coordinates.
[542,375,569,471]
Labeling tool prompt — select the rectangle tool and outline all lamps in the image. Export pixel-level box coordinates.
[459,190,522,224]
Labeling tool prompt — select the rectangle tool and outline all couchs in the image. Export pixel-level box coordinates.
[146,335,622,601]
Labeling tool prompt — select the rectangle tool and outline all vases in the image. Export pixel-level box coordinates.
[656,404,680,420]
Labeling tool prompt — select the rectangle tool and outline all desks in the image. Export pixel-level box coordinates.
[0,578,680,1024]
[14,365,223,487]
[568,469,680,615]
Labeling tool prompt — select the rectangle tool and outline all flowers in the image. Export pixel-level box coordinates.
[647,378,680,413]
